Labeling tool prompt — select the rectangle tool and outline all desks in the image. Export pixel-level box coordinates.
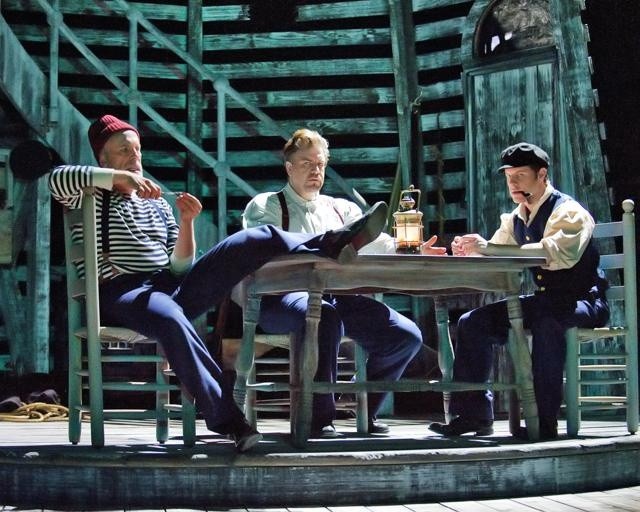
[233,245,549,446]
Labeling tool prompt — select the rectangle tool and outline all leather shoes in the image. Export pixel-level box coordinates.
[367,418,389,432]
[510,424,558,440]
[318,201,388,265]
[430,415,494,436]
[227,418,264,452]
[310,421,336,438]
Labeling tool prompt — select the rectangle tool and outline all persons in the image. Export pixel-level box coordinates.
[240,128,448,438]
[48,114,388,454]
[428,142,610,442]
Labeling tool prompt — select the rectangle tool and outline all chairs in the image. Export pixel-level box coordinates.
[241,322,375,440]
[59,184,201,450]
[508,193,640,439]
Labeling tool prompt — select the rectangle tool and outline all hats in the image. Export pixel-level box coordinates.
[496,143,551,174]
[87,115,141,160]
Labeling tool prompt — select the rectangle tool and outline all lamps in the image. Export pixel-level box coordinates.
[392,184,421,254]
